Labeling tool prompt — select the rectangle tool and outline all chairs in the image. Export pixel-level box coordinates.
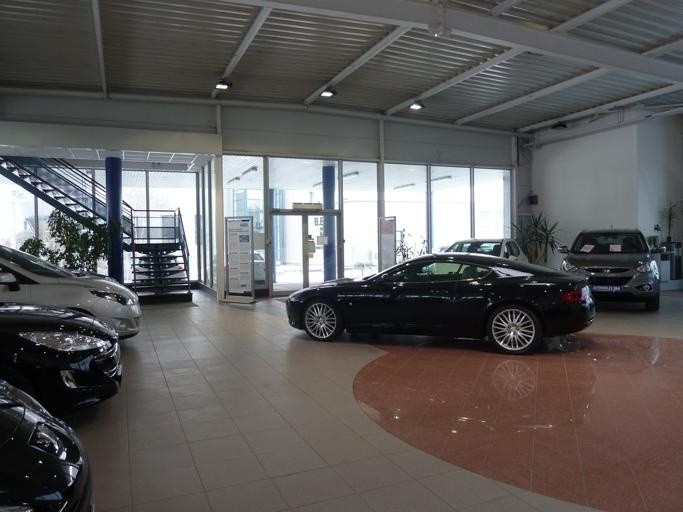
[583,237,635,249]
[467,244,501,255]
[399,265,475,282]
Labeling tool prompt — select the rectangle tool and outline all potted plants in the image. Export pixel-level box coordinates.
[647,197,681,282]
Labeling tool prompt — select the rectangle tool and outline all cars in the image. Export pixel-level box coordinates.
[285,251,597,355]
[0,301,123,411]
[130,255,187,289]
[0,244,142,338]
[558,228,666,312]
[427,238,530,275]
[0,378,95,512]
[207,252,275,296]
